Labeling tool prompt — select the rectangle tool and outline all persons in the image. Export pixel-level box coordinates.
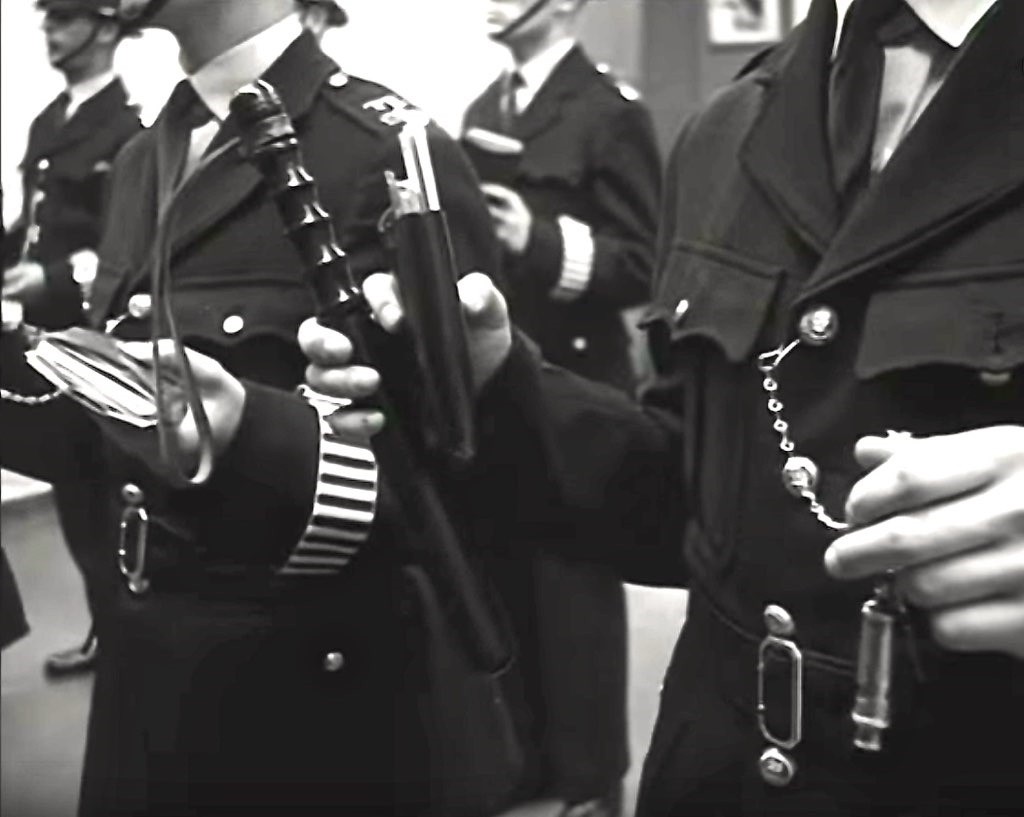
[299,0,1024,817]
[0,0,157,679]
[0,0,559,817]
[460,0,668,817]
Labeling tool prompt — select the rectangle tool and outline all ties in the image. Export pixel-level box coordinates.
[824,0,925,224]
[158,80,215,211]
[53,92,71,133]
[501,72,525,132]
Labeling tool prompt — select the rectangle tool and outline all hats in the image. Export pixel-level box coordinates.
[296,0,349,28]
[37,0,143,37]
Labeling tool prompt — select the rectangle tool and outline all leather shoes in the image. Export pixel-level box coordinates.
[45,635,100,680]
